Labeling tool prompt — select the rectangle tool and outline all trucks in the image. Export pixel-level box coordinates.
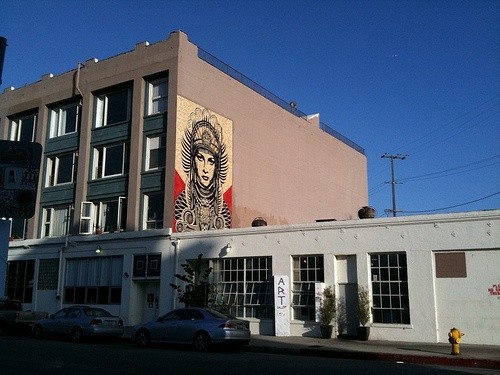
[0,295,49,325]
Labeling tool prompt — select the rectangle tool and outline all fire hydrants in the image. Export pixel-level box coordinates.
[447,327,465,355]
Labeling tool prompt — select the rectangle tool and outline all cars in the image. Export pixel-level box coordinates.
[32,305,126,344]
[132,306,251,353]
